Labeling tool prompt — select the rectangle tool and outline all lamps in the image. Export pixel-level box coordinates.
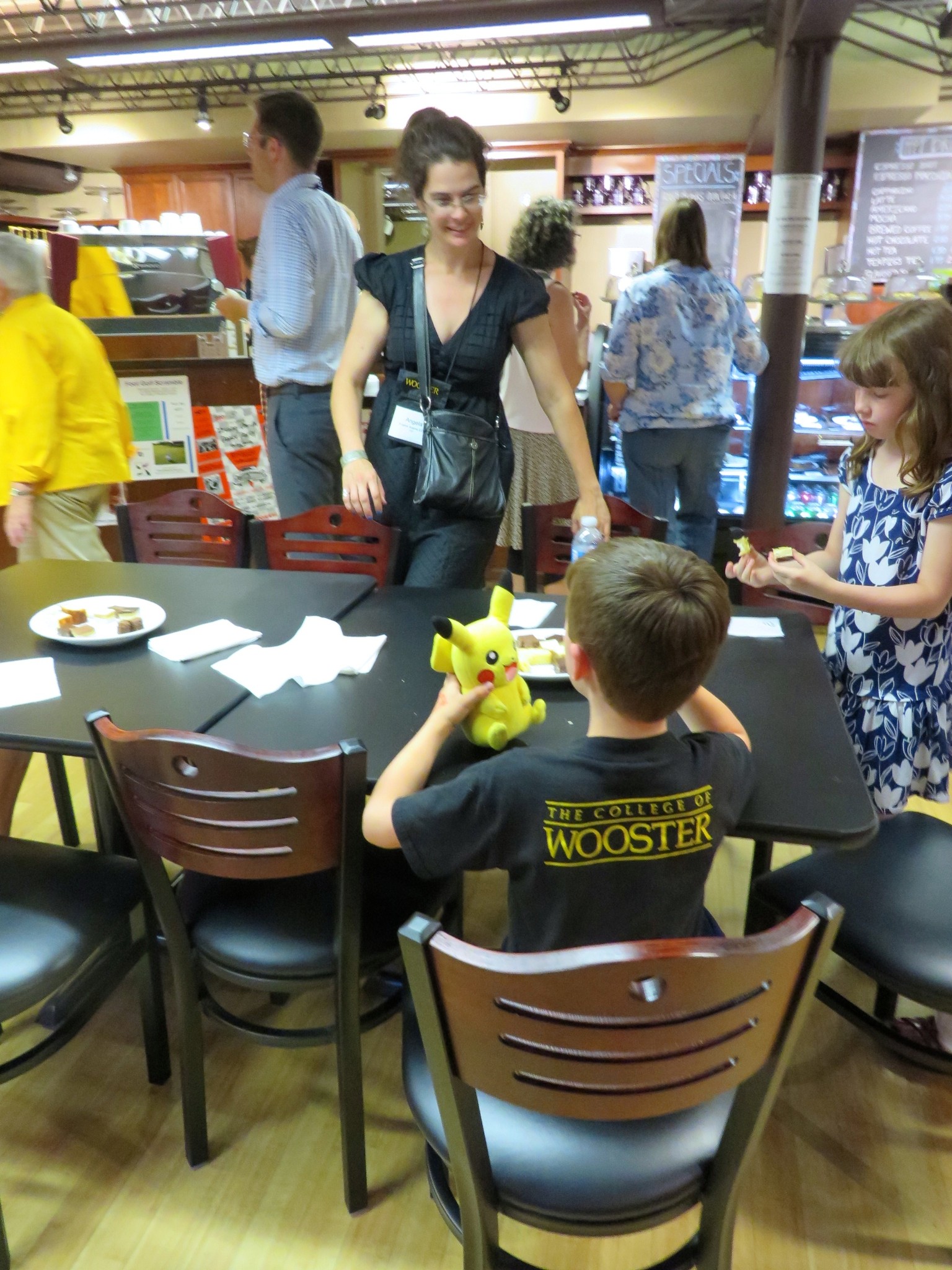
[195,96,214,130]
[363,101,385,121]
[55,111,74,134]
[550,88,571,112]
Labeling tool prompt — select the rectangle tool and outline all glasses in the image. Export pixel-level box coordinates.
[242,131,272,148]
[420,193,487,211]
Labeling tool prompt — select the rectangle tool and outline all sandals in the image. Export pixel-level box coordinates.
[890,1014,948,1051]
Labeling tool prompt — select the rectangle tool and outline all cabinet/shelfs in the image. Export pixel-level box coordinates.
[591,325,874,529]
[563,152,856,215]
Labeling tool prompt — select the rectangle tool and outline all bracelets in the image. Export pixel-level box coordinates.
[339,449,368,468]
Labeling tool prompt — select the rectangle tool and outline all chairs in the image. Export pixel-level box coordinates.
[0,834,174,1270]
[114,489,257,568]
[82,704,450,1215]
[739,518,845,625]
[385,887,844,1269]
[521,496,669,593]
[246,506,407,587]
[755,809,951,1072]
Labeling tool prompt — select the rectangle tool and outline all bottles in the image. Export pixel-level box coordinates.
[570,515,605,565]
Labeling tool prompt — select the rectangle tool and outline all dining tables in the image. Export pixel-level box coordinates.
[198,587,880,935]
[2,557,381,860]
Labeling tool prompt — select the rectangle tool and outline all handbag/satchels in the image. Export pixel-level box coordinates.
[413,408,507,520]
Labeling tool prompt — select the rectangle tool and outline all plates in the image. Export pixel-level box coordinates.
[509,624,571,682]
[30,594,166,646]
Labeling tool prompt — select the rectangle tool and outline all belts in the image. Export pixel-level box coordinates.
[266,384,332,397]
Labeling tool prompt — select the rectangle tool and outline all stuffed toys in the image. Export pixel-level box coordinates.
[429,568,548,751]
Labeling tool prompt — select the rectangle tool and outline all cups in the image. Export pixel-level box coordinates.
[53,207,207,263]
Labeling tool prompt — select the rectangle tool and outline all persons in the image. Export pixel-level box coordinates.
[599,199,772,566]
[0,233,136,565]
[492,201,591,593]
[67,246,135,320]
[362,539,756,953]
[329,105,610,591]
[725,298,952,828]
[212,88,368,574]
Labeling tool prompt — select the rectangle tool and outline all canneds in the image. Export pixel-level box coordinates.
[571,167,844,207]
[785,482,838,520]
